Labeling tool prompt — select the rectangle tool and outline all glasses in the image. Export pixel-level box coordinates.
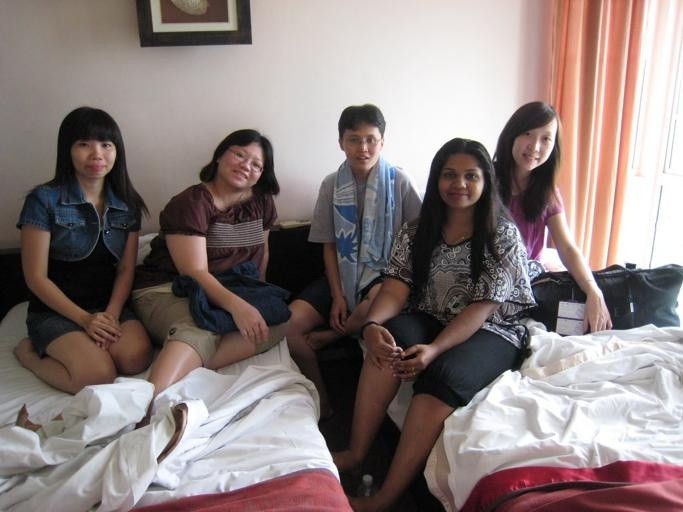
[343,134,382,146]
[230,149,264,171]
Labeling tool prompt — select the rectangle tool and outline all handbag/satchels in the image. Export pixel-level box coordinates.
[530,263,682,336]
[172,262,291,335]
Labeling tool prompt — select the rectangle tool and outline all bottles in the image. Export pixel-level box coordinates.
[356,474,379,498]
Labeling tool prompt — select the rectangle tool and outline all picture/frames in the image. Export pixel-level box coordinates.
[133,1,256,51]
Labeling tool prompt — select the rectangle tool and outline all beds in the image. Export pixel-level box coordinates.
[357,236,683,512]
[0,226,358,511]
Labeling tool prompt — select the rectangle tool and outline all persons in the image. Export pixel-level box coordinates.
[327,136,538,511]
[284,105,423,424]
[14,106,155,395]
[131,127,293,433]
[490,102,614,337]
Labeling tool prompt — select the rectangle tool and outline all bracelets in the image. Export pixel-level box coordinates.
[358,321,378,340]
